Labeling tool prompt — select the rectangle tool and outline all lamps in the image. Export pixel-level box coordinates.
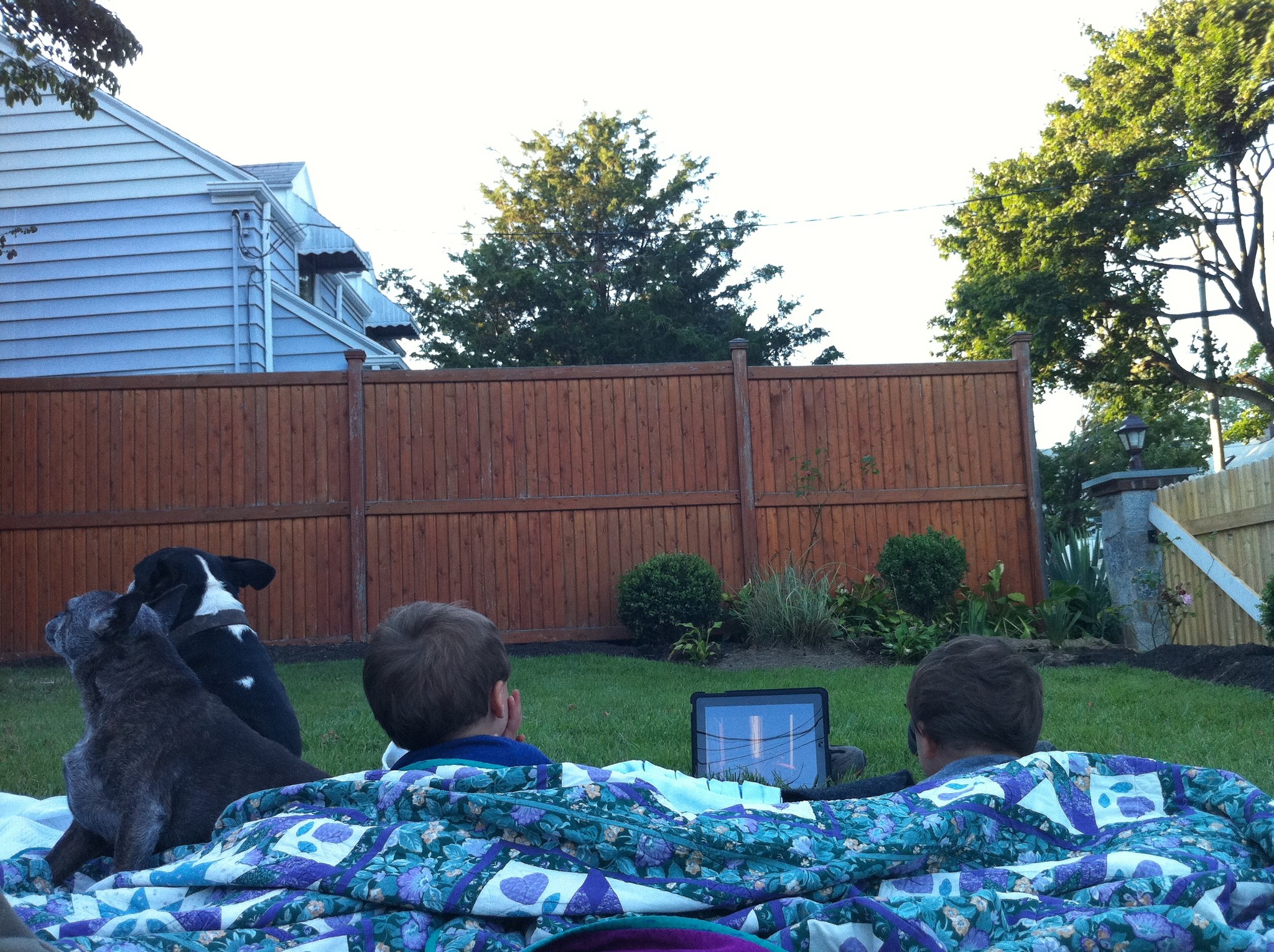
[1114,413,1151,472]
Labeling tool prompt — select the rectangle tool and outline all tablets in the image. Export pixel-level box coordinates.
[690,688,831,789]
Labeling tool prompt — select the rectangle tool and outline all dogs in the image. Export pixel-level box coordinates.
[46,547,334,891]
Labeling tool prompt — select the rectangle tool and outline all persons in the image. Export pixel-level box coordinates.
[361,600,549,771]
[908,635,1045,775]
